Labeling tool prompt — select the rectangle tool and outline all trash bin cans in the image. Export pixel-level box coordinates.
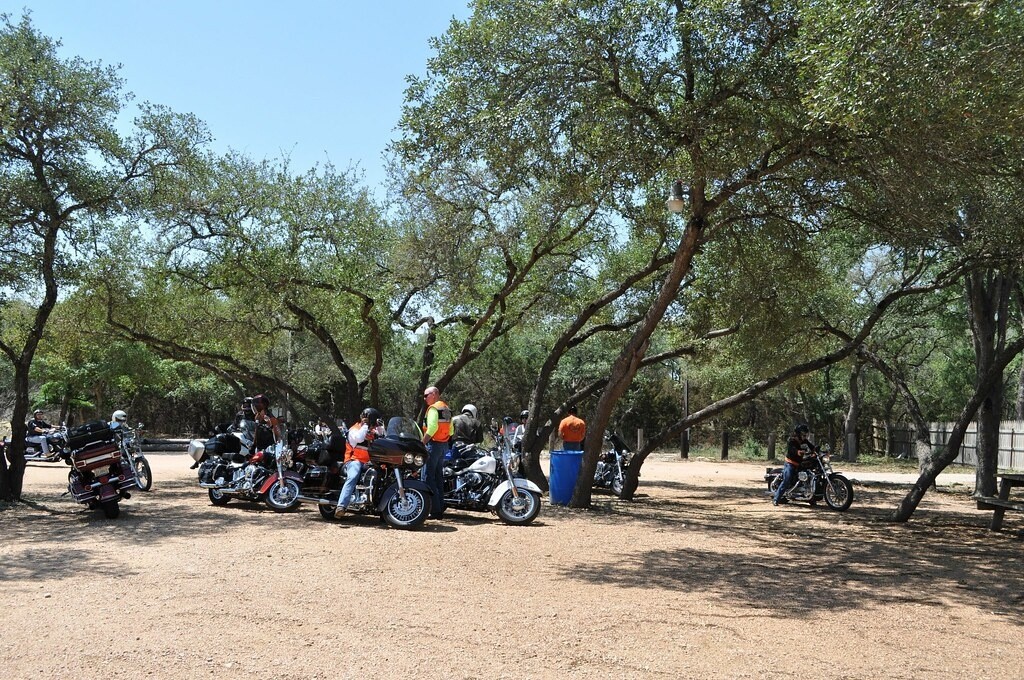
[549,449,584,506]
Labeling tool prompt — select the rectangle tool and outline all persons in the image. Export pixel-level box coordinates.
[557,405,586,451]
[27,408,61,457]
[489,409,529,478]
[213,395,288,457]
[771,424,818,507]
[106,409,146,438]
[421,387,452,520]
[304,416,349,445]
[334,408,387,519]
[448,404,485,461]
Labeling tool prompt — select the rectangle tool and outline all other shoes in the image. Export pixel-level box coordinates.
[426,512,443,520]
[335,506,346,518]
[773,500,777,506]
[45,452,54,457]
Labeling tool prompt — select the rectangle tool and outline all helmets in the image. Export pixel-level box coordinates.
[241,396,253,410]
[520,410,529,421]
[504,416,512,424]
[461,403,478,419]
[794,424,810,441]
[569,405,577,414]
[250,394,271,411]
[111,409,127,421]
[359,407,379,426]
[33,409,43,418]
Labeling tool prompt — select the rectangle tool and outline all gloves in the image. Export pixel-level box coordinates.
[376,418,385,427]
[58,426,64,429]
[43,431,48,435]
[360,410,371,425]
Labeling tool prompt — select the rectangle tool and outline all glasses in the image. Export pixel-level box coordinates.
[522,416,528,420]
[425,392,433,398]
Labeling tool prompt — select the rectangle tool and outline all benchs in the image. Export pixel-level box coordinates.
[973,497,1024,511]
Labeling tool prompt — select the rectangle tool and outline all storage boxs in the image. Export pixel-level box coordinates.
[66,419,113,451]
[205,435,241,456]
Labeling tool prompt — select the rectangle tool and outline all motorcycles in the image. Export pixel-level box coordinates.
[59,421,137,518]
[185,415,544,531]
[3,421,70,463]
[591,431,641,496]
[108,421,153,492]
[765,442,854,513]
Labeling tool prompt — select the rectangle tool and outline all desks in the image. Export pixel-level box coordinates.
[990,474,1024,533]
